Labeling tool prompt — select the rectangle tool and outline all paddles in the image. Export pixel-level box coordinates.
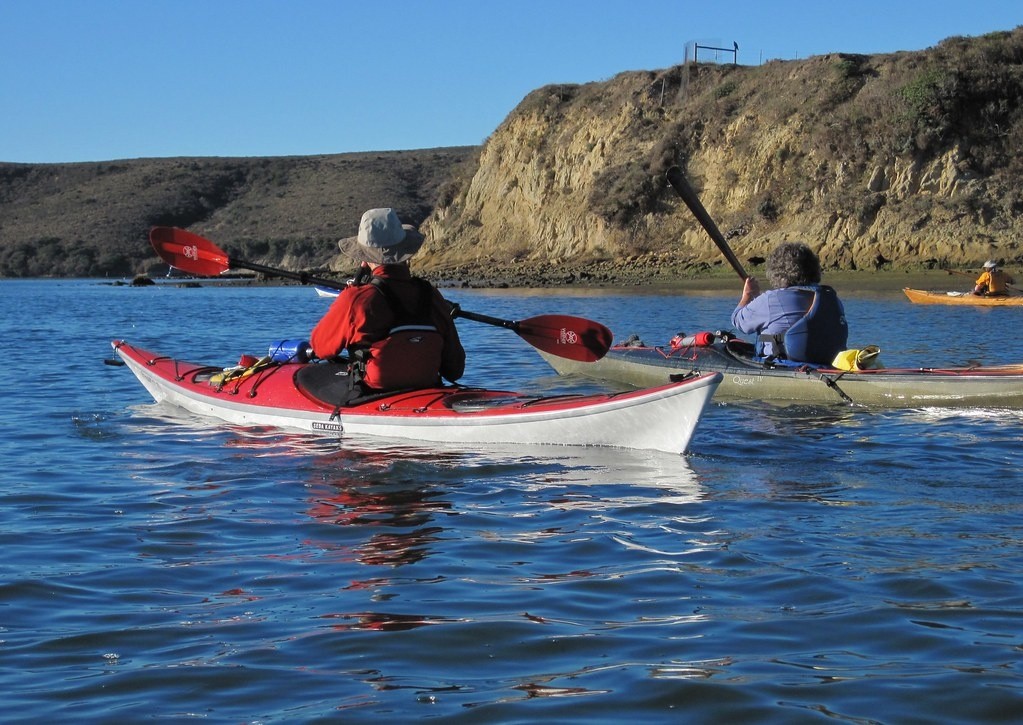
[943,267,1023,293]
[664,165,749,284]
[148,224,613,363]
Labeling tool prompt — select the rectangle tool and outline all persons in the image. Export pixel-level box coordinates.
[975,259,1015,296]
[297,207,467,408]
[729,242,848,368]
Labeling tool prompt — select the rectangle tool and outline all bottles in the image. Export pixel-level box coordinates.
[675,331,717,346]
[269,339,315,364]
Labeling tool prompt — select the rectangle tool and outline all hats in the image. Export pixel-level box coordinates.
[982,260,996,268]
[338,206,425,265]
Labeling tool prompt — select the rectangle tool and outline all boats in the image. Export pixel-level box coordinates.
[534,330,1023,409]
[102,329,725,460]
[903,286,1023,309]
